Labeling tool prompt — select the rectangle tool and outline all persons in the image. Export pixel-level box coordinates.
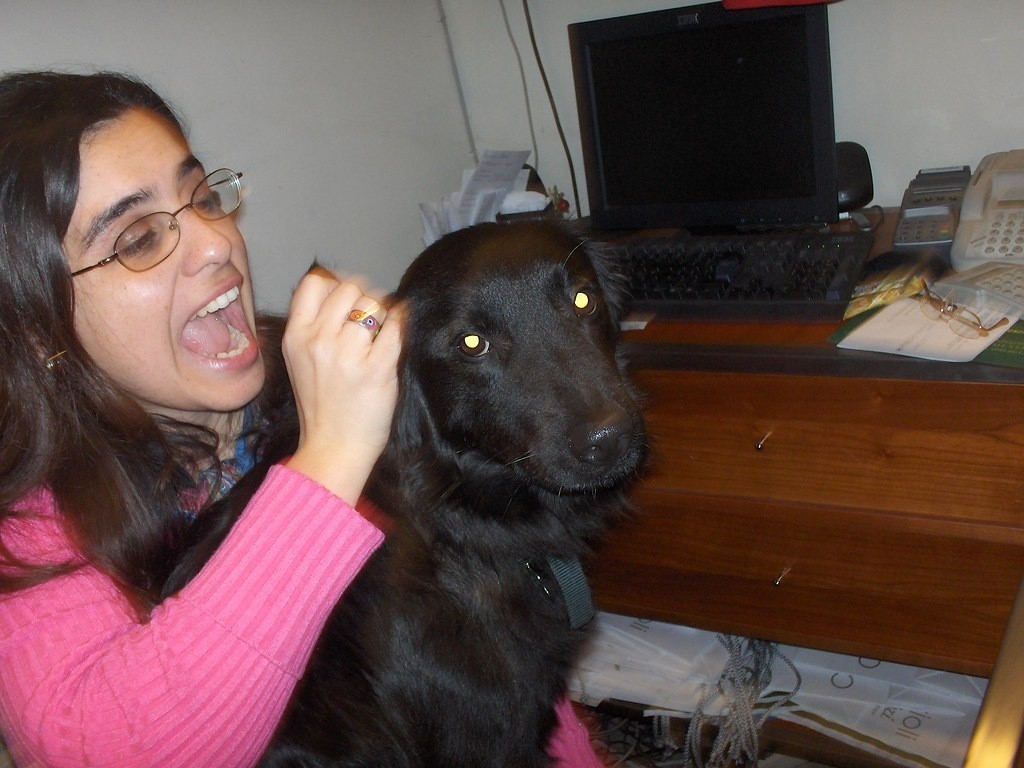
[1,71,607,768]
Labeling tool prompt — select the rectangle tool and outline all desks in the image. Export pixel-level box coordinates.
[588,208,1024,768]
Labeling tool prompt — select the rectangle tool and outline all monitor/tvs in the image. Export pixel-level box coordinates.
[568,0,841,242]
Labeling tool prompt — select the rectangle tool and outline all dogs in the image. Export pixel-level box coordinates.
[155,216,652,768]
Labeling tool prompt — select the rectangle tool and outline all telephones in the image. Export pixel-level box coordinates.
[933,146,1024,273]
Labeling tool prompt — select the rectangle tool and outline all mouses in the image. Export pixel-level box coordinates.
[859,248,948,283]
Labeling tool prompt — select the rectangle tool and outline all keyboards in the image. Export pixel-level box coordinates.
[613,230,873,317]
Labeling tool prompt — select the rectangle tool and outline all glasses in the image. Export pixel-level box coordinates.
[69,168,245,277]
[919,278,1009,340]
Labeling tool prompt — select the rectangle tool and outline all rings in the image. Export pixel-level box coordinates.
[346,309,381,336]
